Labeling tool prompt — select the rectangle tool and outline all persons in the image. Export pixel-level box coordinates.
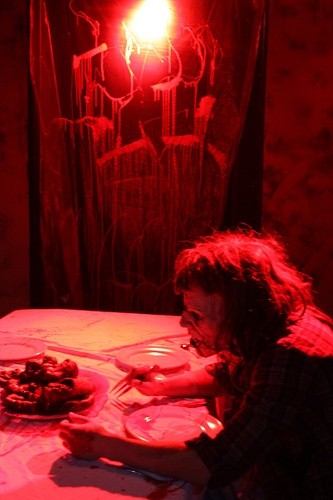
[57,230,333,500]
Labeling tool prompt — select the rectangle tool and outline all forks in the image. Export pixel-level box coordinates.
[109,365,161,400]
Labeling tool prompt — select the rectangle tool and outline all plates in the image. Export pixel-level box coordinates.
[0,337,47,360]
[116,344,190,369]
[125,405,225,443]
[0,368,111,420]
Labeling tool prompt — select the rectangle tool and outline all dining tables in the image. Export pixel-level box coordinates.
[0,308,240,500]
